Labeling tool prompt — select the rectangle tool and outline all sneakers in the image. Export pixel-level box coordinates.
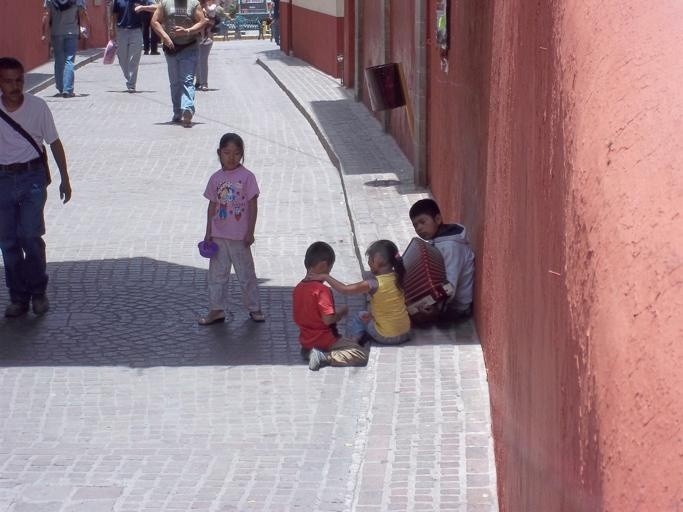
[64,91,75,98]
[203,38,213,45]
[183,110,192,127]
[308,347,324,370]
[129,88,135,92]
[172,110,182,121]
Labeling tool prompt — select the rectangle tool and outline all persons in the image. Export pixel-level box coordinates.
[266,1,277,18]
[216,0,235,23]
[309,240,413,345]
[409,198,476,322]
[42,0,92,98]
[201,133,266,325]
[255,17,272,39]
[267,12,274,25]
[151,0,207,127]
[195,0,217,91]
[0,57,71,317]
[108,0,158,93]
[294,241,368,370]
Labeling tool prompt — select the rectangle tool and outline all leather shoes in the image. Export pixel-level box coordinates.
[202,85,208,91]
[31,295,51,316]
[5,302,27,318]
[249,312,266,323]
[198,310,225,324]
